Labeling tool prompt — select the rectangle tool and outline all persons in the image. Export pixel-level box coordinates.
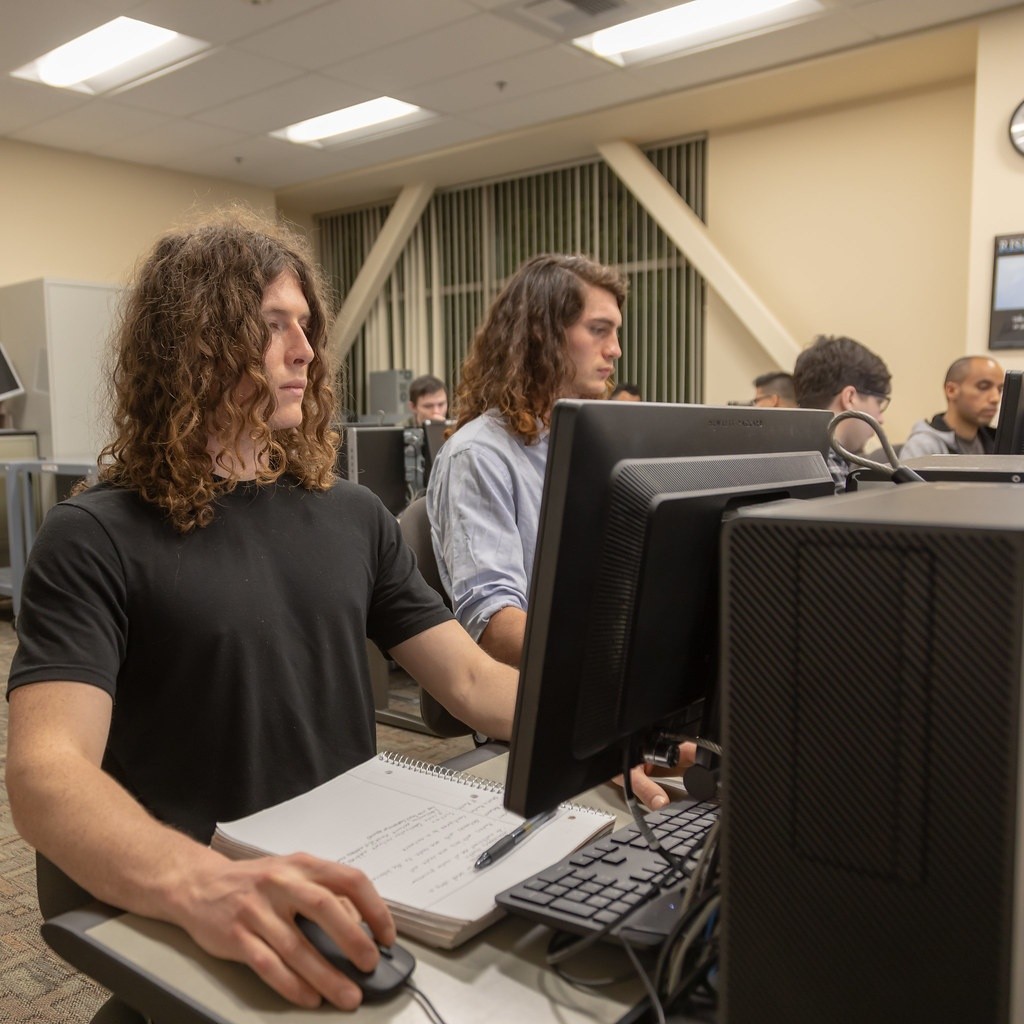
[898,354,1005,463]
[395,373,450,429]
[609,384,644,404]
[752,332,893,495]
[422,249,627,676]
[5,221,700,1011]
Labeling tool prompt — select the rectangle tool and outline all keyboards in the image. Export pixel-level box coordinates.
[495,796,718,952]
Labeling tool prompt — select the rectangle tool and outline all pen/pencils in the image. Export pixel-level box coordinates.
[473,805,559,873]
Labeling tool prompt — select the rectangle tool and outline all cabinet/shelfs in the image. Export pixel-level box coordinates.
[1,277,134,463]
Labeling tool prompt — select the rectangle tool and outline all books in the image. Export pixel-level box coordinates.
[211,747,617,953]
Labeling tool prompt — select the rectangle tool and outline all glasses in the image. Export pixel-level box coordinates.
[832,387,892,412]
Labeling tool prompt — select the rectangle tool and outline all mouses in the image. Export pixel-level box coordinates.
[294,912,416,999]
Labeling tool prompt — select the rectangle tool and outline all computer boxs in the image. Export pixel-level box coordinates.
[884,452,1024,482]
[716,482,1024,1024]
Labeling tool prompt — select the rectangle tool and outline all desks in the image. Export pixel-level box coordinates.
[40,740,688,1024]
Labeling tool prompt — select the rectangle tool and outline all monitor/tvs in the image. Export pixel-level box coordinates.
[424,398,834,820]
[993,369,1024,455]
